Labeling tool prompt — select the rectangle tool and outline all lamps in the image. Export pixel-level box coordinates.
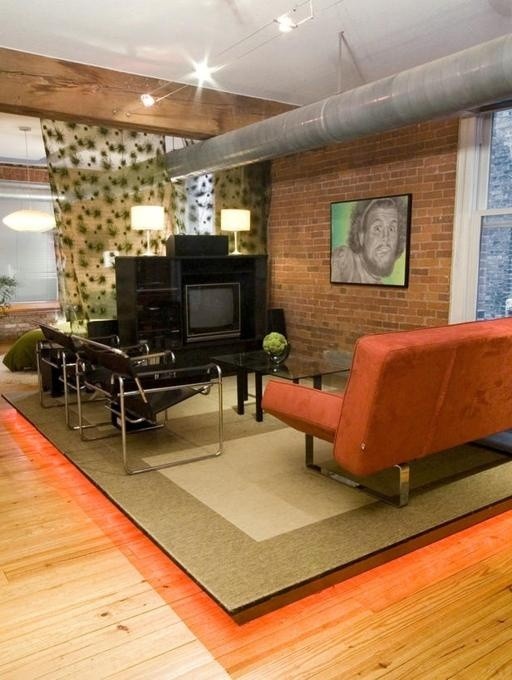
[129,205,166,257]
[220,208,251,255]
[2,126,57,234]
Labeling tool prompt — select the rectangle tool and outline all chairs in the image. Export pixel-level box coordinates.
[68,333,225,477]
[31,318,151,430]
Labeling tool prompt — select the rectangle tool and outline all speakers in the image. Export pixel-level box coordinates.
[269,309,287,339]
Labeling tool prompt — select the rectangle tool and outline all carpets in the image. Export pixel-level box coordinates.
[1,369,512,617]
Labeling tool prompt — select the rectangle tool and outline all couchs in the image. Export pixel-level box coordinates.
[261,316,512,509]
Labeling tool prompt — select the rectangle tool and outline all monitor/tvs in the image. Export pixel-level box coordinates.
[184,283,242,342]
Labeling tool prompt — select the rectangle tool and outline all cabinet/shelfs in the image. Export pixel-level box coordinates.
[113,256,184,348]
[165,332,270,387]
